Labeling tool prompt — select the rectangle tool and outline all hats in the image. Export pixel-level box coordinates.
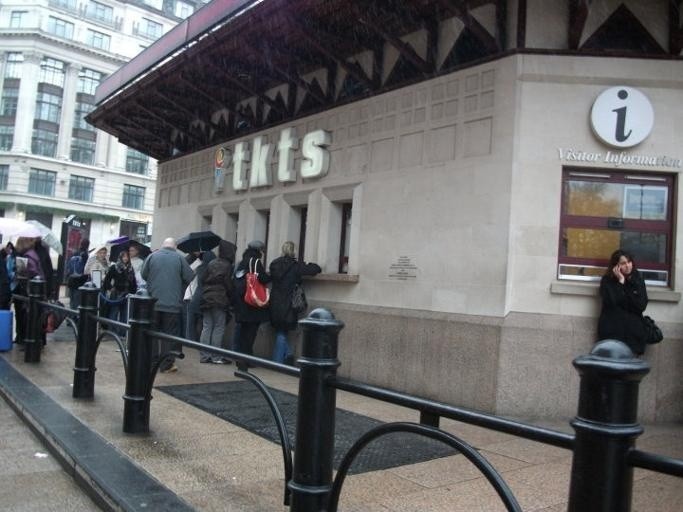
[247,239,266,251]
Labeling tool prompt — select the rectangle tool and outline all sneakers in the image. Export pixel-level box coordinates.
[159,364,178,374]
[213,357,233,366]
[198,357,213,363]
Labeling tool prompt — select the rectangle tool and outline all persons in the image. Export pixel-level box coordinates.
[598,249,648,357]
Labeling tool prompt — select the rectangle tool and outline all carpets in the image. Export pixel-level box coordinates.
[152,376,480,476]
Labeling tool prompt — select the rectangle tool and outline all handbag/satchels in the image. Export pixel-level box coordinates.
[642,315,664,345]
[290,282,307,313]
[242,256,270,310]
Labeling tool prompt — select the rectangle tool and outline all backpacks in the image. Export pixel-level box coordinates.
[67,252,86,279]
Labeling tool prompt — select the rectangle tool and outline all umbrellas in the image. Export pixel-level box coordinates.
[176,230,223,252]
[28,220,63,256]
[0,217,42,239]
